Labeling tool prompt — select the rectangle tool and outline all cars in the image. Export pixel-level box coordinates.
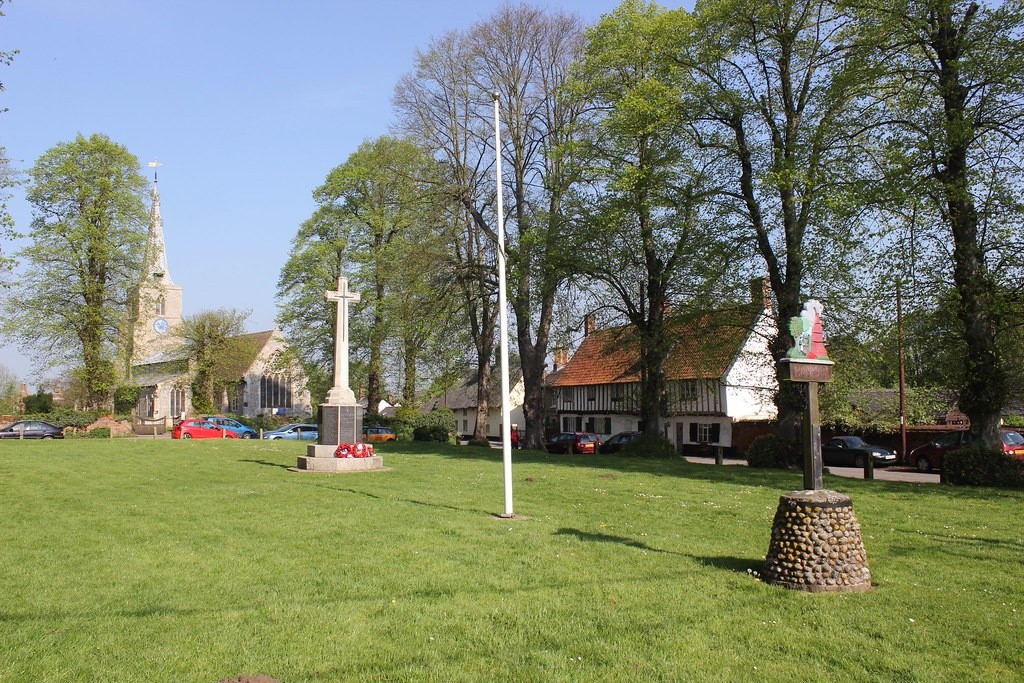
[820,435,899,467]
[361,426,399,442]
[206,415,258,439]
[908,430,1024,474]
[0,420,65,441]
[257,421,319,441]
[548,430,602,454]
[600,430,645,453]
[171,417,241,439]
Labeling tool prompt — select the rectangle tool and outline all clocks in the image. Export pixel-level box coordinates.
[153,319,169,333]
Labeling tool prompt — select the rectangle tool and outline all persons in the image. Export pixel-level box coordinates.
[221,421,225,425]
[512,430,519,450]
[594,437,600,455]
